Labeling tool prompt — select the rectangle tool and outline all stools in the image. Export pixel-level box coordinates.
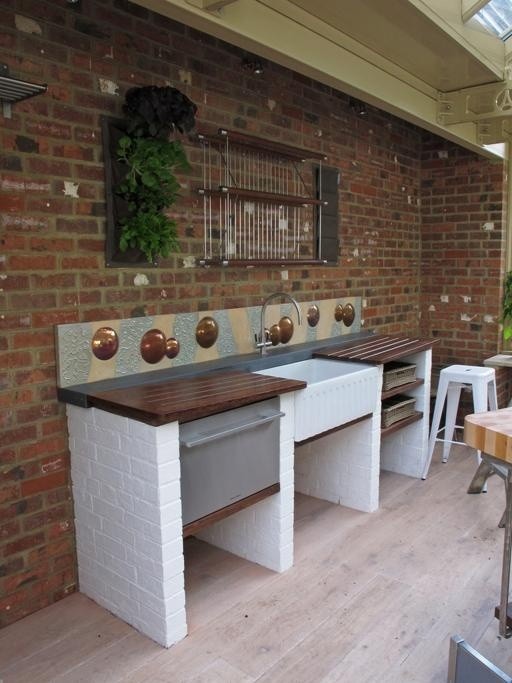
[423,364,498,478]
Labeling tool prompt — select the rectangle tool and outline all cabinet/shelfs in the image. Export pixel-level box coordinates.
[311,334,440,513]
[57,361,306,649]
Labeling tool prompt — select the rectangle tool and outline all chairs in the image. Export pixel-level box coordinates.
[448,635,512,683]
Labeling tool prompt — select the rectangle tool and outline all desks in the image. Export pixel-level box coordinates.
[463,406,512,640]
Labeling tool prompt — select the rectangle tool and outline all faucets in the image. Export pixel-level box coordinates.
[261,293,302,355]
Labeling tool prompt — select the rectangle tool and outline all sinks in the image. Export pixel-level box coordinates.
[252,358,379,442]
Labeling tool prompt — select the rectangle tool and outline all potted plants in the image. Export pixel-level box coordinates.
[113,83,198,263]
